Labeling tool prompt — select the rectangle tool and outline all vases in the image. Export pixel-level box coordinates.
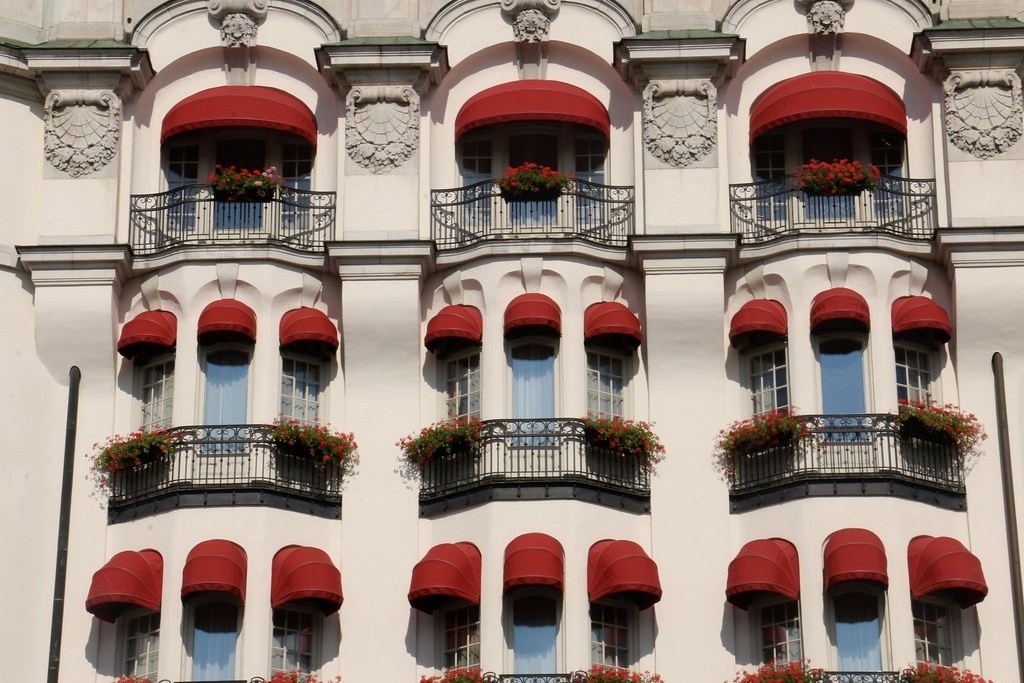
[500,183,562,203]
[130,443,162,463]
[804,180,866,196]
[740,428,793,455]
[903,415,957,446]
[588,428,637,455]
[276,438,322,459]
[213,184,274,203]
[429,436,470,459]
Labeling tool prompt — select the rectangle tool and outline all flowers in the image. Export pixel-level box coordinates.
[394,408,484,465]
[264,411,360,479]
[893,397,987,453]
[114,657,993,683]
[494,161,576,196]
[713,405,828,480]
[580,411,666,476]
[789,157,880,195]
[205,163,289,202]
[86,421,185,493]
